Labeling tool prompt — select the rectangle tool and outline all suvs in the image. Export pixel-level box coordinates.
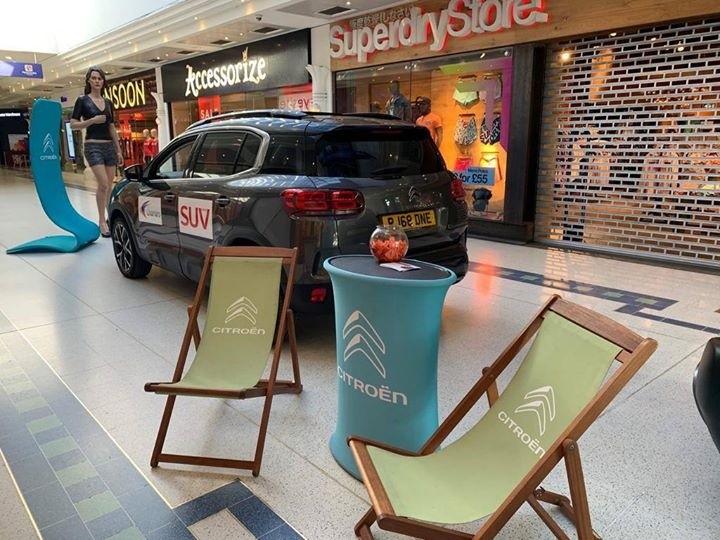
[105,107,469,346]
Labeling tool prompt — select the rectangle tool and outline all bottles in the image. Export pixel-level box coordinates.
[369,225,409,262]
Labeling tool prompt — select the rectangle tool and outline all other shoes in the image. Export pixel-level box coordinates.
[98,225,111,237]
[106,219,109,226]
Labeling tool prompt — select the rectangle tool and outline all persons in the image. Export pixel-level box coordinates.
[385,80,413,122]
[69,66,123,237]
[141,127,159,166]
[414,96,443,148]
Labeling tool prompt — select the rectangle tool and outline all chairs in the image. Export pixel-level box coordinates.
[347,295,657,539]
[144,245,303,477]
